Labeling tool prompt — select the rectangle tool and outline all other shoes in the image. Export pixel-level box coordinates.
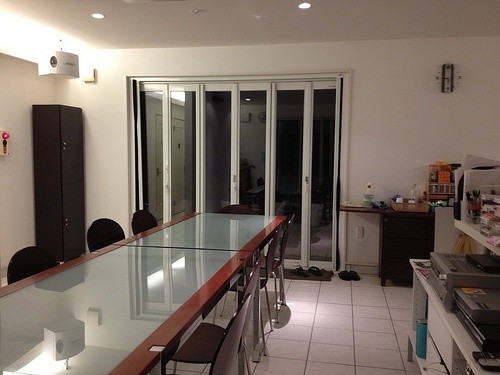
[348,270,361,281]
[338,270,351,281]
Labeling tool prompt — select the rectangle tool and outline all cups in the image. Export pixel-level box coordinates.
[465,196,482,224]
[397,197,403,203]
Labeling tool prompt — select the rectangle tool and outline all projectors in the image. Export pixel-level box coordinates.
[37,51,79,78]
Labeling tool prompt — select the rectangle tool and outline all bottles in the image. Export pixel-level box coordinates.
[363,184,375,208]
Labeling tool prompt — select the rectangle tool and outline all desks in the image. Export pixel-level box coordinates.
[453,218,500,256]
[0,211,288,375]
[407,259,500,375]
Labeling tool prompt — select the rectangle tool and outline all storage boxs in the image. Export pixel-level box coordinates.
[426,163,462,206]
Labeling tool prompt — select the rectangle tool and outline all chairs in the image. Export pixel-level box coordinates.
[169,214,296,375]
[7,210,157,285]
[217,204,250,319]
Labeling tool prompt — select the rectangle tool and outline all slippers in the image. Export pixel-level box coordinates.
[291,267,309,278]
[308,266,323,276]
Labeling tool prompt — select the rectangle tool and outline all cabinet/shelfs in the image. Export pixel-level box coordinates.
[32,104,86,262]
[340,201,436,287]
